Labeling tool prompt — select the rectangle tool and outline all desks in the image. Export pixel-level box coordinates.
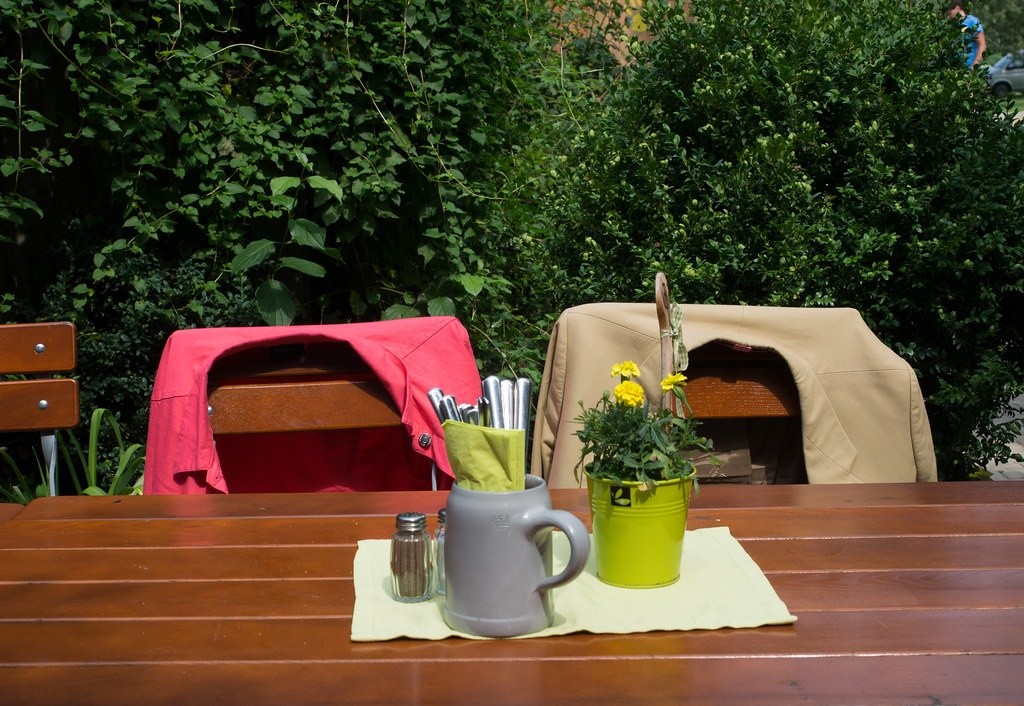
[0,481,1024,706]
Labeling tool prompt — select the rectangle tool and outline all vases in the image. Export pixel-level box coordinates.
[583,458,697,589]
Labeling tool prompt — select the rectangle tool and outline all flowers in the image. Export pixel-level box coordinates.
[567,360,724,497]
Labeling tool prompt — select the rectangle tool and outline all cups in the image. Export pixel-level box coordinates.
[442,474,591,638]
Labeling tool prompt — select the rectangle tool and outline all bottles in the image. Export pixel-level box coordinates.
[390,511,435,604]
[432,507,447,596]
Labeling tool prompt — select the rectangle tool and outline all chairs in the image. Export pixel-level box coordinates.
[0,322,79,496]
[144,317,482,495]
[529,302,937,483]
